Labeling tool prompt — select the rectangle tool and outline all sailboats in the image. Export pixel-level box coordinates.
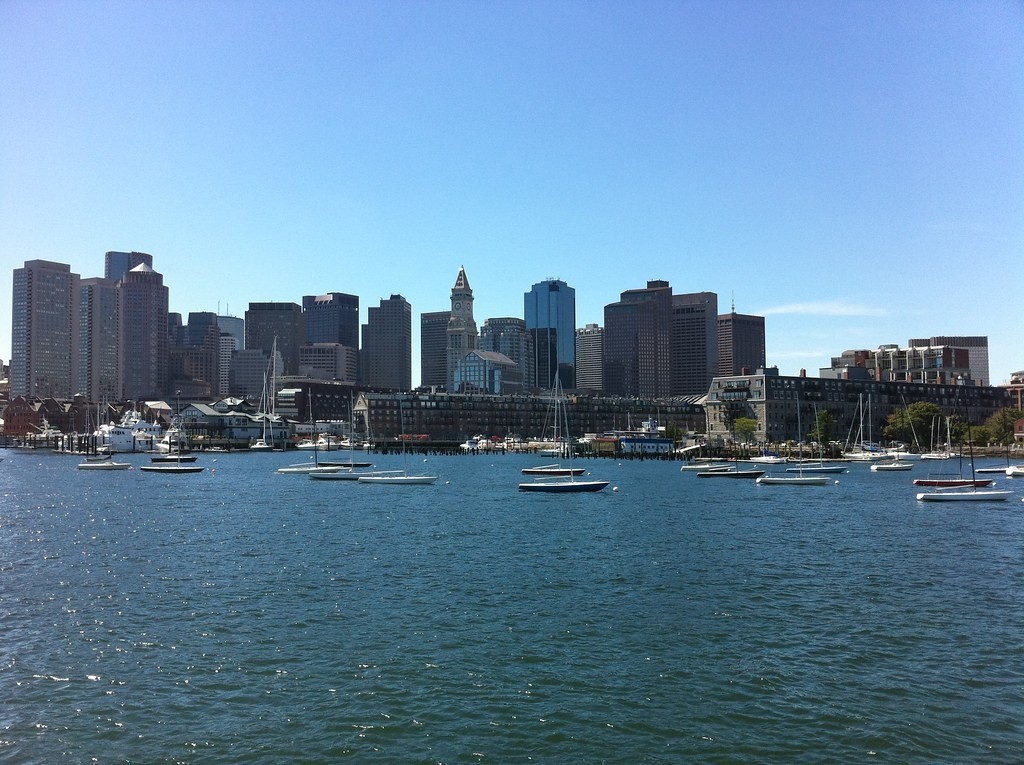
[518,380,610,491]
[841,393,956,461]
[141,391,205,472]
[681,407,736,472]
[786,402,847,473]
[756,393,832,485]
[522,401,585,475]
[870,404,914,470]
[278,387,438,483]
[697,410,765,478]
[78,395,131,469]
[913,383,1024,502]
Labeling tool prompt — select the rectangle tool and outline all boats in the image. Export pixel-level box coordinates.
[26,405,190,454]
[750,456,786,464]
[250,372,375,452]
[796,463,821,468]
[695,458,727,462]
